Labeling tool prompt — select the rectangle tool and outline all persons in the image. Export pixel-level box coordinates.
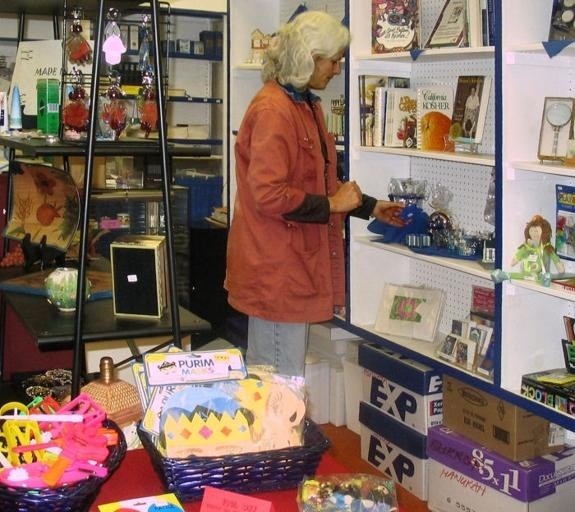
[224,10,411,378]
[462,86,480,137]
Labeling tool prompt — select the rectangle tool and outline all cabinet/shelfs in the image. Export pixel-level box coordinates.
[499,1,575,434]
[1,1,223,298]
[346,0,497,395]
[228,1,347,243]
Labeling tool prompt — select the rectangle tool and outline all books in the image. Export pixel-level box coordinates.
[358,75,453,151]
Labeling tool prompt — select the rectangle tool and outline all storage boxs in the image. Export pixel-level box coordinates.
[358,338,575,512]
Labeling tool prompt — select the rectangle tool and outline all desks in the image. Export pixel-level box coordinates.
[15,296,210,363]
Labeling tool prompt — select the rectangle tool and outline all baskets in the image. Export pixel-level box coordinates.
[1,415,127,512]
[135,418,332,502]
